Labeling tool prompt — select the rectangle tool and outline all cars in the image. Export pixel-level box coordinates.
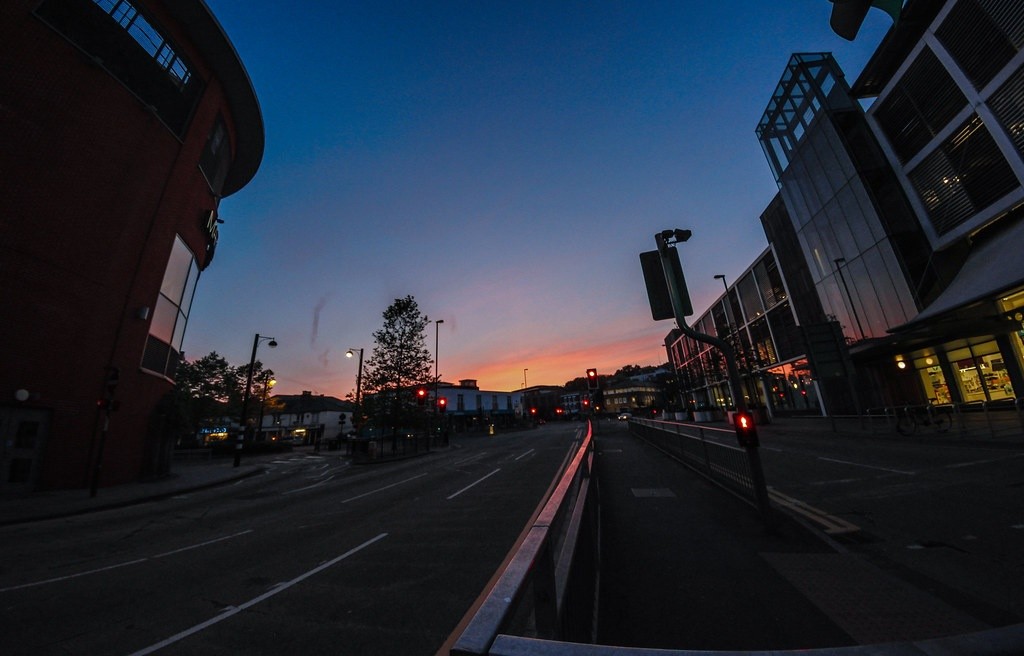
[618,413,632,421]
[537,419,546,425]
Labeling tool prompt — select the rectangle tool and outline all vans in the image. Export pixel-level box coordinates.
[279,436,303,447]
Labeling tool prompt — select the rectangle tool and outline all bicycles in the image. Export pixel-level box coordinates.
[896,398,952,437]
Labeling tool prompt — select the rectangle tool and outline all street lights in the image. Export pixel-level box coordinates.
[346,348,364,419]
[713,274,758,405]
[435,319,445,412]
[524,368,529,387]
[833,257,866,338]
[234,334,278,463]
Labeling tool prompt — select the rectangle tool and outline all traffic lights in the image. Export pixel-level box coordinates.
[584,401,588,406]
[531,407,536,414]
[438,398,447,413]
[733,412,758,446]
[96,365,120,410]
[586,369,600,390]
[653,408,657,415]
[557,409,563,413]
[417,388,425,405]
[595,405,599,412]
